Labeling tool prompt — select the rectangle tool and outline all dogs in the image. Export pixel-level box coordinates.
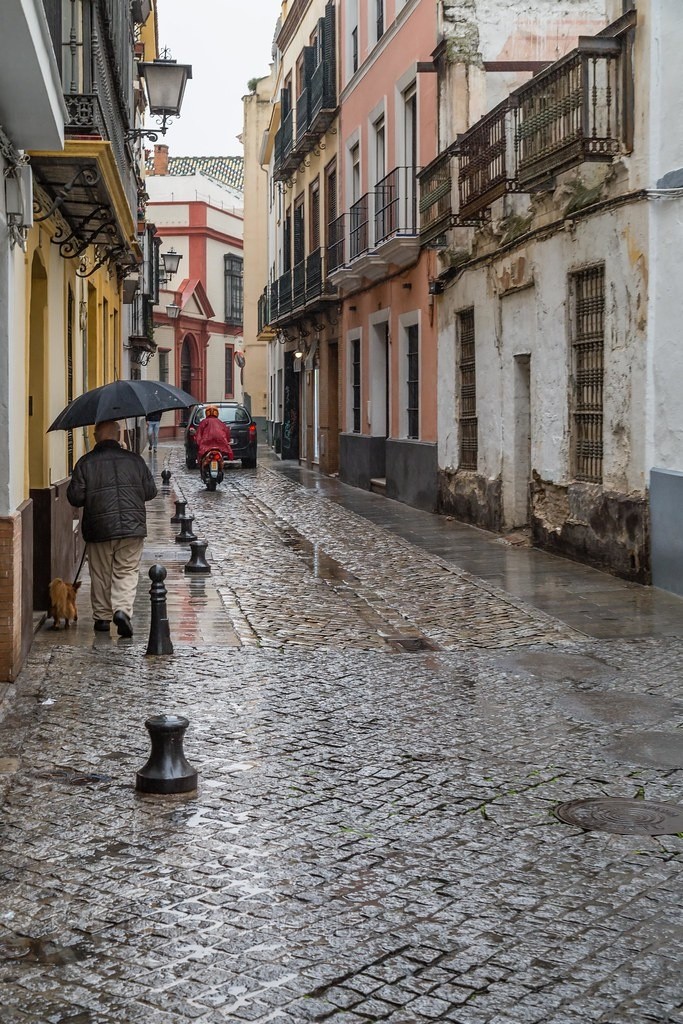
[48,575,84,630]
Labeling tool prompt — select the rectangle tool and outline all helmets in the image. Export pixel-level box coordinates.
[205,406,218,417]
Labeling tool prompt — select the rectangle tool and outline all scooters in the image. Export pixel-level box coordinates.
[189,423,237,492]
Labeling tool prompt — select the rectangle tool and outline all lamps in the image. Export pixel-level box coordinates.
[293,337,311,360]
[125,46,192,141]
[158,247,182,282]
[153,303,182,328]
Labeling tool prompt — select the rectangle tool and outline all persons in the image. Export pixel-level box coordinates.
[145,412,162,452]
[191,405,230,479]
[66,419,158,638]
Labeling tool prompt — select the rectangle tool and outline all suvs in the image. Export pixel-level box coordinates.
[179,402,258,469]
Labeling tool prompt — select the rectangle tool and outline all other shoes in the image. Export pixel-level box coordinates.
[149,444,152,450]
[113,611,133,637]
[94,621,110,631]
[154,449,157,452]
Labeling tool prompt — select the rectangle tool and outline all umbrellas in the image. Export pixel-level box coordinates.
[46,367,203,452]
